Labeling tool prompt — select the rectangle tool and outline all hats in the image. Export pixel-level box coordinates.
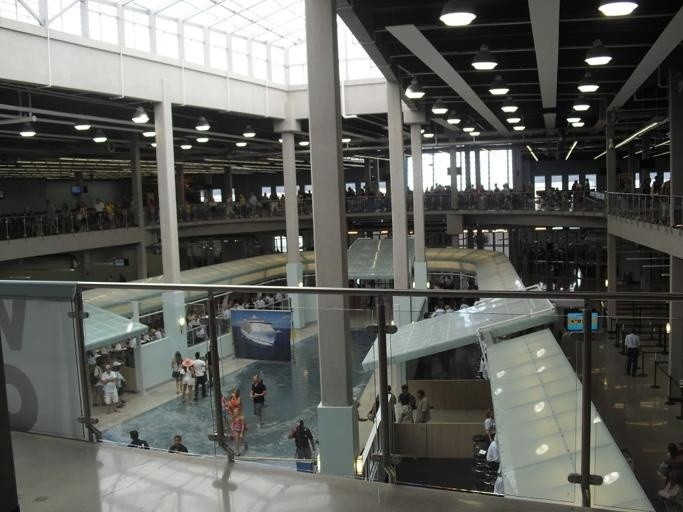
[112,361,121,366]
[105,365,110,369]
[182,359,193,367]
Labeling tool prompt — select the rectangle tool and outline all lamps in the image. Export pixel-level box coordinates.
[131,101,150,125]
[195,114,210,131]
[93,123,107,143]
[197,136,209,142]
[20,99,35,138]
[278,137,283,144]
[152,141,157,148]
[342,135,352,144]
[236,142,247,147]
[242,123,257,138]
[143,126,155,138]
[181,142,192,151]
[400,1,643,143]
[75,100,92,132]
[299,136,310,147]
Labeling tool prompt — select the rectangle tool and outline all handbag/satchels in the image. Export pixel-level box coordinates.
[171,370,179,377]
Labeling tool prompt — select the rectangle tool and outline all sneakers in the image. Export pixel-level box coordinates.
[106,400,126,414]
[236,444,248,457]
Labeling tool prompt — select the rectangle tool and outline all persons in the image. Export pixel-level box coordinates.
[84,290,315,459]
[353,275,505,495]
[624,329,641,377]
[658,443,683,511]
